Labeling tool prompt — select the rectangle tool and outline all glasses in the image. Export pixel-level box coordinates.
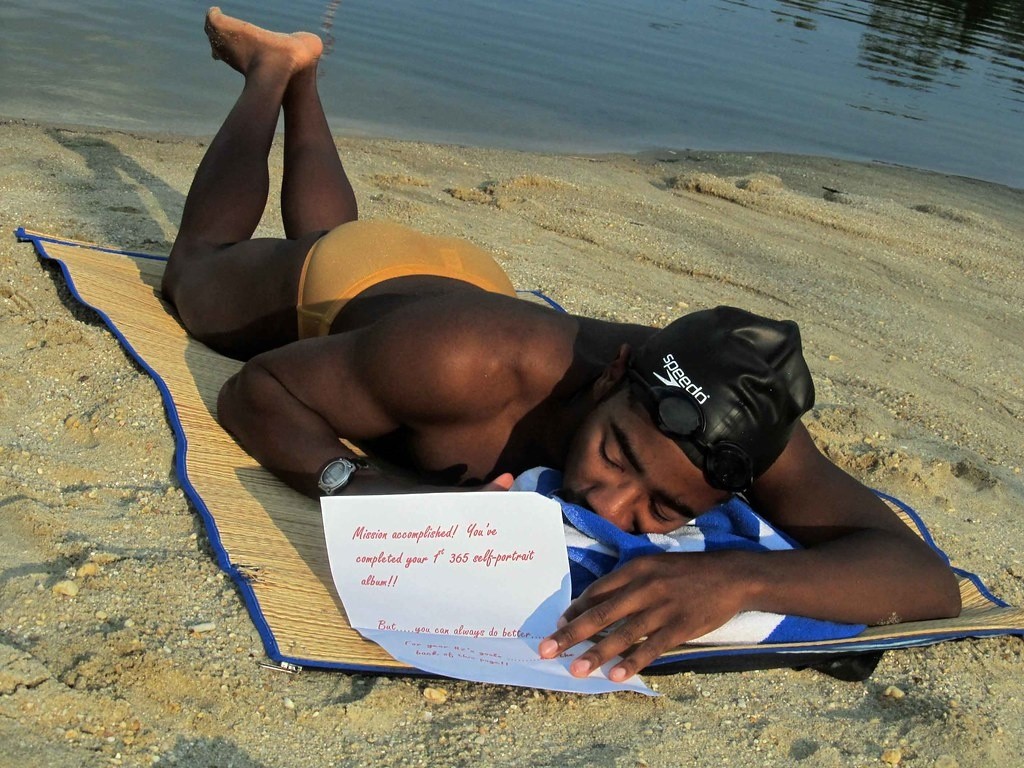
[626,366,755,492]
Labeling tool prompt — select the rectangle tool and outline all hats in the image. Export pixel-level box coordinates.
[626,305,815,491]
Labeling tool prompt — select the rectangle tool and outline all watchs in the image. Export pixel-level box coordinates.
[317,441,364,497]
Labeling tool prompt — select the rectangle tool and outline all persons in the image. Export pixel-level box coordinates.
[159,8,964,684]
